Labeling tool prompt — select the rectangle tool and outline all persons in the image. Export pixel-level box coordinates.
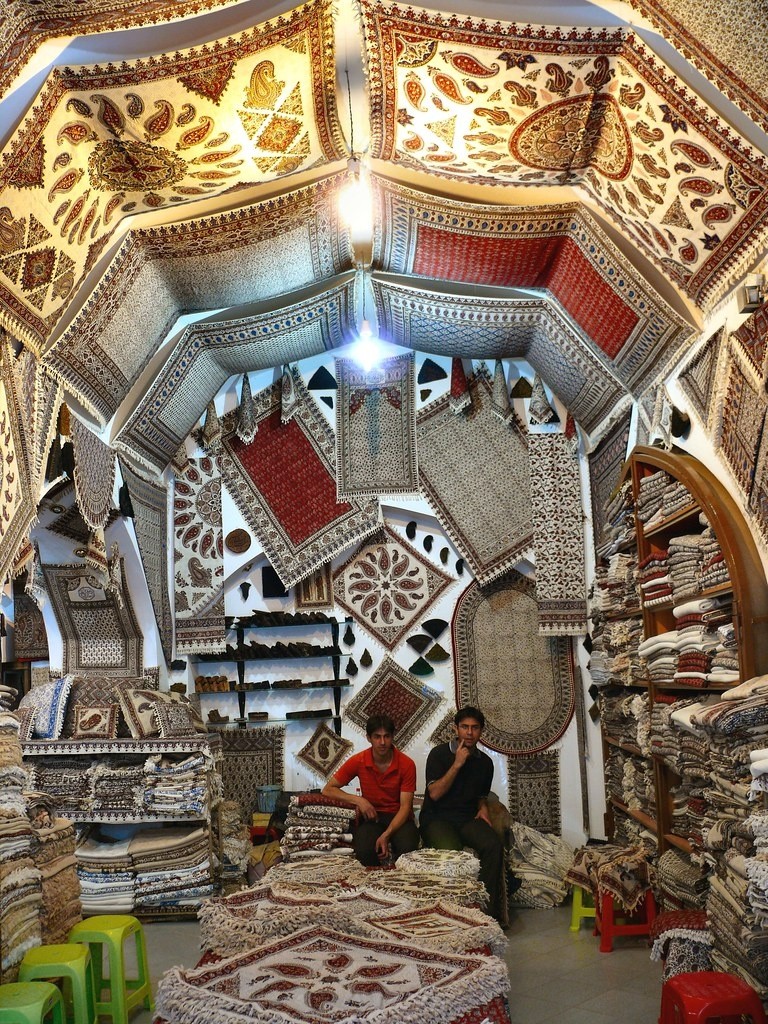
[420,706,506,920]
[321,714,420,868]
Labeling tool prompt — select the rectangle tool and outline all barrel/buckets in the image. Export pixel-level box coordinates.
[255,783,283,815]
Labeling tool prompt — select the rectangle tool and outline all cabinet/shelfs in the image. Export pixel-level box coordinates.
[193,609,356,737]
[595,444,768,963]
[22,733,225,926]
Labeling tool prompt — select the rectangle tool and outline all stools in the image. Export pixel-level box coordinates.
[594,864,655,952]
[0,981,66,1024]
[17,945,98,1024]
[248,826,278,846]
[70,914,153,1024]
[571,861,598,933]
[659,971,765,1024]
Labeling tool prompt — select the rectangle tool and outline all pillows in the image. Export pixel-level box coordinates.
[75,705,117,739]
[153,704,196,738]
[62,678,149,739]
[13,709,35,739]
[17,675,76,740]
[117,686,207,738]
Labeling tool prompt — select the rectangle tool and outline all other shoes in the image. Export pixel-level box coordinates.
[489,914,507,929]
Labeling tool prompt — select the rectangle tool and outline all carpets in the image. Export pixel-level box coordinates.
[0,0,768,1024]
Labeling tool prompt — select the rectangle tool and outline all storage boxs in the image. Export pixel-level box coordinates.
[252,811,274,827]
